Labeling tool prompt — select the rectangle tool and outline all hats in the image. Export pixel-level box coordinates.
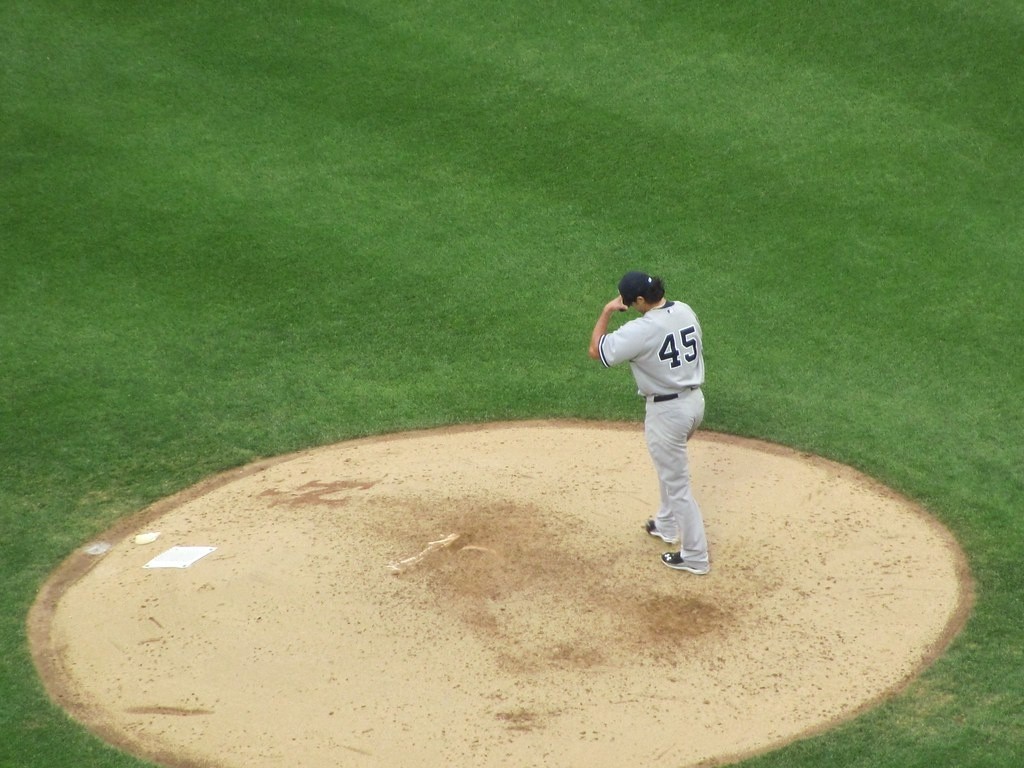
[619,269,656,313]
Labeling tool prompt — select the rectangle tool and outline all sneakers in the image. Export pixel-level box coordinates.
[660,551,710,576]
[644,519,678,545]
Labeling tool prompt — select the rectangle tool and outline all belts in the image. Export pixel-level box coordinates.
[644,393,679,403]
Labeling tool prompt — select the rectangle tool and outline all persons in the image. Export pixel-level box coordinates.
[589,271,710,575]
